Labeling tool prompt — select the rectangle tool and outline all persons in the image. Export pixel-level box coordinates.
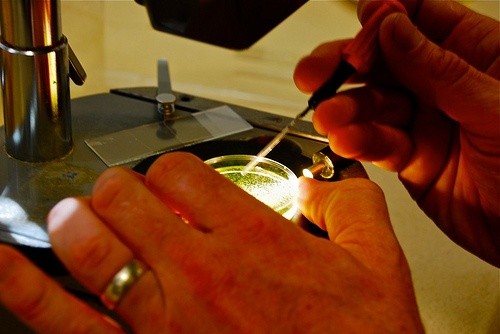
[1,0,500,334]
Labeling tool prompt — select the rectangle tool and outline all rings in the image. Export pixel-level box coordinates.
[98,256,151,312]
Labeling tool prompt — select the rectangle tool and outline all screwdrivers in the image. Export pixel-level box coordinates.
[239,0,408,176]
